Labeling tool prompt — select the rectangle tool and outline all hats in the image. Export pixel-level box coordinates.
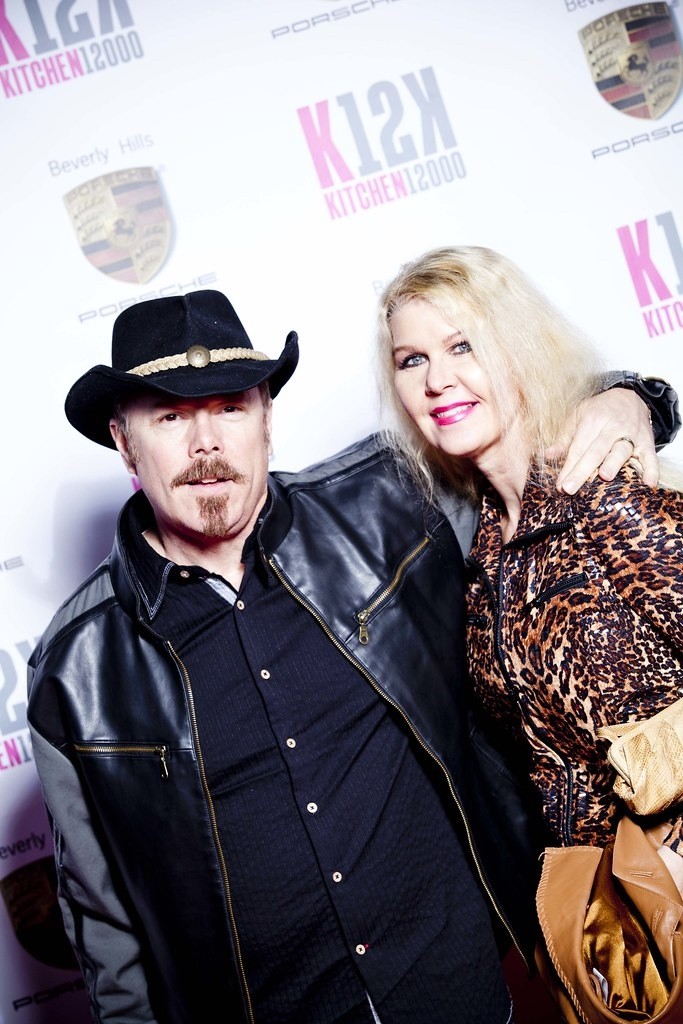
[64,290,302,451]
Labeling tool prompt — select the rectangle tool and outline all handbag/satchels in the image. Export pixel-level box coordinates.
[535,824,683,1024]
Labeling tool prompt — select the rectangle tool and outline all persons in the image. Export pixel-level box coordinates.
[374,245,683,902]
[26,291,680,1024]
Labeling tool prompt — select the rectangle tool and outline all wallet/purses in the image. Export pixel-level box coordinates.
[598,700,683,816]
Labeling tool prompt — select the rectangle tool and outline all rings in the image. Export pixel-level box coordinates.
[619,437,634,447]
